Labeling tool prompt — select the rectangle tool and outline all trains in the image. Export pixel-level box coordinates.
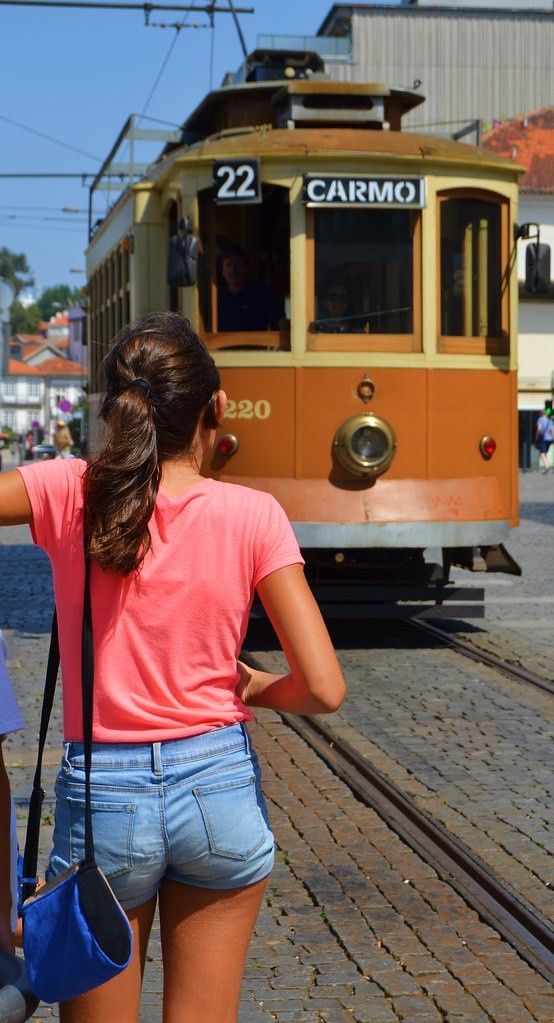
[85,49,539,624]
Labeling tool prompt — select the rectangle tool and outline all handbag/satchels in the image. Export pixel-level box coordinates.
[17,859,135,1003]
[536,439,548,452]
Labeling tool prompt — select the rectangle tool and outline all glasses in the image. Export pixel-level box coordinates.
[327,293,348,303]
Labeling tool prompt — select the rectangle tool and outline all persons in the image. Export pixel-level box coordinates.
[0,635,23,957]
[535,408,554,474]
[209,245,283,331]
[316,281,367,333]
[1,310,348,1023]
[54,419,75,460]
